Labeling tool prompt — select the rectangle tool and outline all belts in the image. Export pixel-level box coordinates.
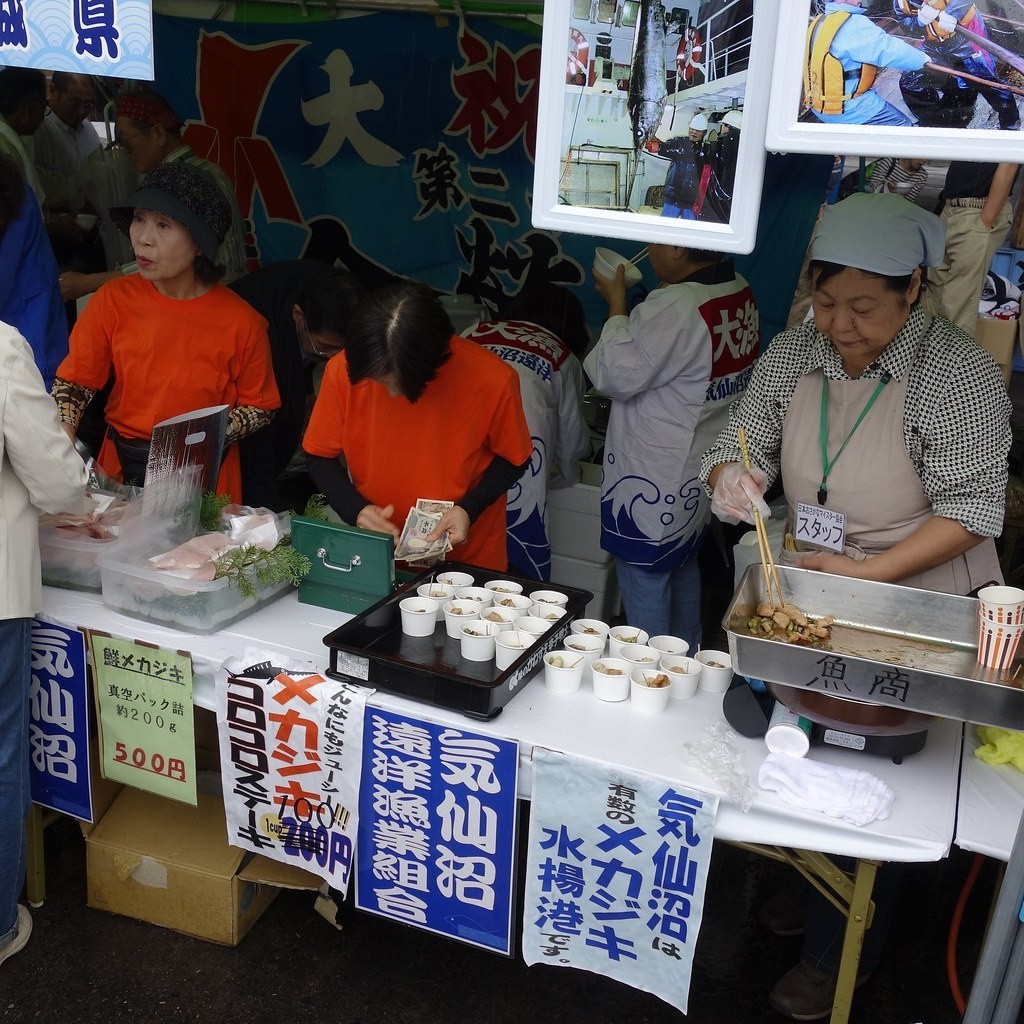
[945,197,987,209]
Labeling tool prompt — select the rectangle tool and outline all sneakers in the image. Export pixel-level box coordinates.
[759,879,808,935]
[768,949,872,1020]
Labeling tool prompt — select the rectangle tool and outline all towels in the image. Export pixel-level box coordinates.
[756,753,895,828]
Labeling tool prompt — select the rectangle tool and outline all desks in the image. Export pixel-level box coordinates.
[1000,475,1024,586]
[952,722,1023,954]
[26,586,962,1024]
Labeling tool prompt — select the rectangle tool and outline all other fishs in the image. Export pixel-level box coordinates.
[625,0,668,149]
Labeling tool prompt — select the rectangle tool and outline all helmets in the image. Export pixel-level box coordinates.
[718,110,743,129]
[689,112,708,131]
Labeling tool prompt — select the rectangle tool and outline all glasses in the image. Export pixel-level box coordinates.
[116,127,149,152]
[33,104,51,119]
[61,93,94,110]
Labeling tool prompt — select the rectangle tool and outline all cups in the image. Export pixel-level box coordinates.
[977,586,1024,671]
[398,572,568,671]
[544,619,734,713]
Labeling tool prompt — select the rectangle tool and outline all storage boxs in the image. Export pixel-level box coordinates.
[290,517,419,616]
[39,512,299,635]
[975,293,1024,394]
[77,706,343,947]
[544,460,624,624]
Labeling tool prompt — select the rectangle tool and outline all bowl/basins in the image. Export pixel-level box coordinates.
[594,247,643,289]
[896,182,914,197]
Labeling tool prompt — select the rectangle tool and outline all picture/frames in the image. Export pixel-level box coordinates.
[763,0,1024,162]
[531,0,781,254]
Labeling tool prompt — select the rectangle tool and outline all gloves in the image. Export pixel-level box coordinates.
[938,11,957,33]
[918,2,940,25]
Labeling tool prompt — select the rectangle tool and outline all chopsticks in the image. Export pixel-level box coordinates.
[737,427,785,609]
[624,246,650,271]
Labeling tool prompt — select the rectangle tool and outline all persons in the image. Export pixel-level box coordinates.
[840,158,929,204]
[59,93,251,303]
[798,0,1021,134]
[301,281,533,570]
[698,192,1013,1018]
[0,318,100,969]
[645,108,742,224]
[457,290,591,583]
[221,257,370,514]
[916,161,1021,340]
[581,243,761,659]
[0,66,71,393]
[45,161,281,507]
[20,71,96,325]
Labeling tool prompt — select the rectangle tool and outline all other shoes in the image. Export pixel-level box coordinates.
[0,903,33,966]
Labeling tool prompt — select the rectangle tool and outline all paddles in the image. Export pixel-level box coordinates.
[925,62,1024,97]
[908,0,1024,76]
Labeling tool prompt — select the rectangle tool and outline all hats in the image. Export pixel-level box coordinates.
[109,163,234,264]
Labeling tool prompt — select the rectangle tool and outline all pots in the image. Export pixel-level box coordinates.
[764,683,950,765]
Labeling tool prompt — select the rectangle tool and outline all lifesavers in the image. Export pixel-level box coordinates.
[675,26,702,81]
[566,27,588,75]
